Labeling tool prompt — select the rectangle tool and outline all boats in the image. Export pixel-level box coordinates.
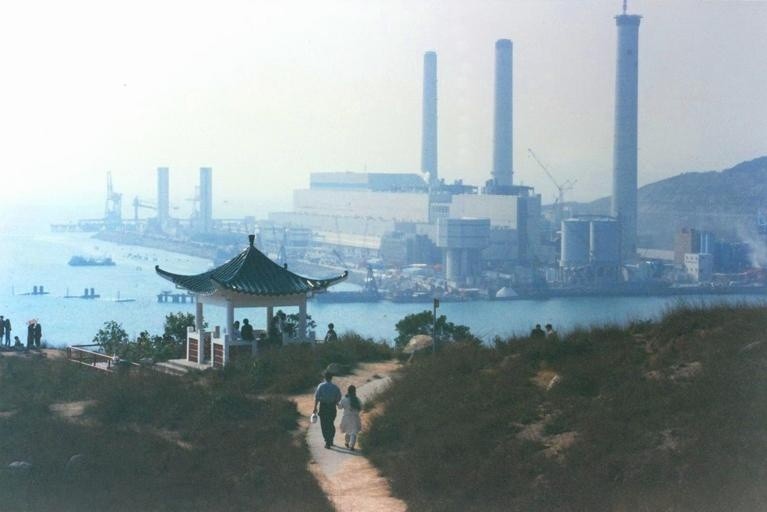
[316,265,380,303]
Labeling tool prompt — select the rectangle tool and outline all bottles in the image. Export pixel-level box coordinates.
[311,411,317,423]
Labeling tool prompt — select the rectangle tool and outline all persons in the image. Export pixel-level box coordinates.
[324,323,337,342]
[532,324,545,336]
[280,314,289,345]
[232,321,240,341]
[272,310,282,336]
[338,385,364,449]
[546,324,556,338]
[313,371,341,448]
[241,318,254,340]
[0,315,41,348]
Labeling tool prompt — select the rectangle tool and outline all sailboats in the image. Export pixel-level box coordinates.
[68,240,116,265]
